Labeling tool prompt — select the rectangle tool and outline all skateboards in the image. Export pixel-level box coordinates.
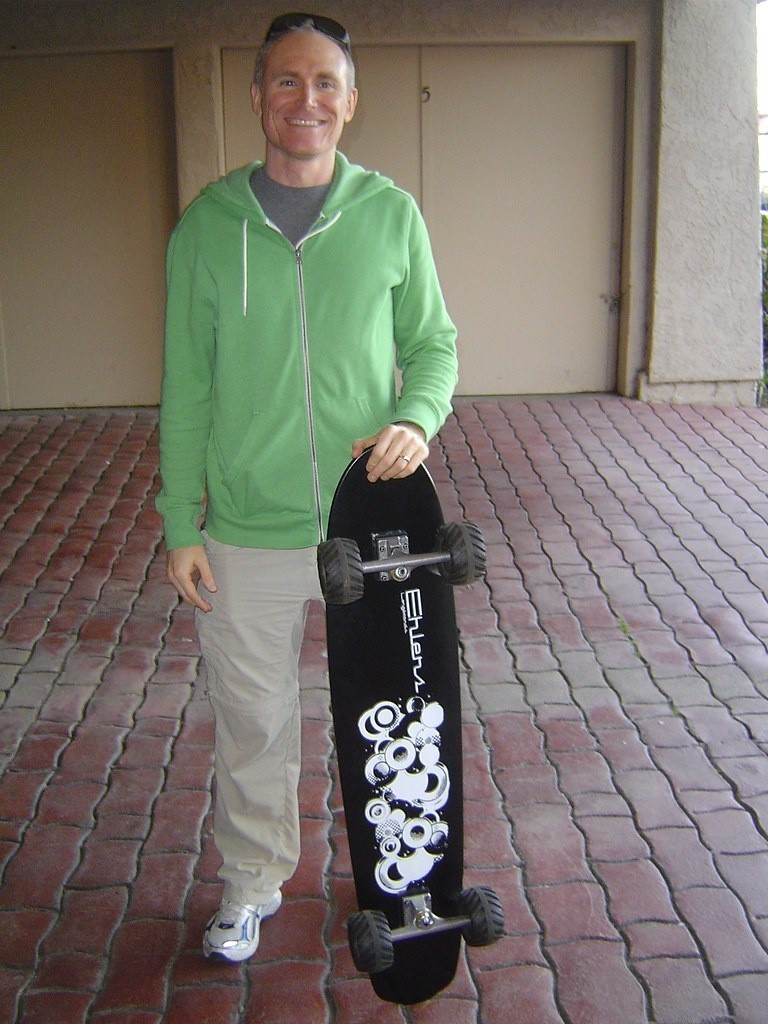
[316,442,506,1007]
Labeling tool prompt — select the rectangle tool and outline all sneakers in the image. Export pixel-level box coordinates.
[202,892,284,962]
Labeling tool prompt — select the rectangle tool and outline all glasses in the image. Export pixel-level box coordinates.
[264,13,351,54]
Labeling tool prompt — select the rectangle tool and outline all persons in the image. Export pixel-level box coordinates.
[153,13,460,963]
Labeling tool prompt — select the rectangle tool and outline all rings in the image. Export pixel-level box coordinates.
[400,454,411,463]
